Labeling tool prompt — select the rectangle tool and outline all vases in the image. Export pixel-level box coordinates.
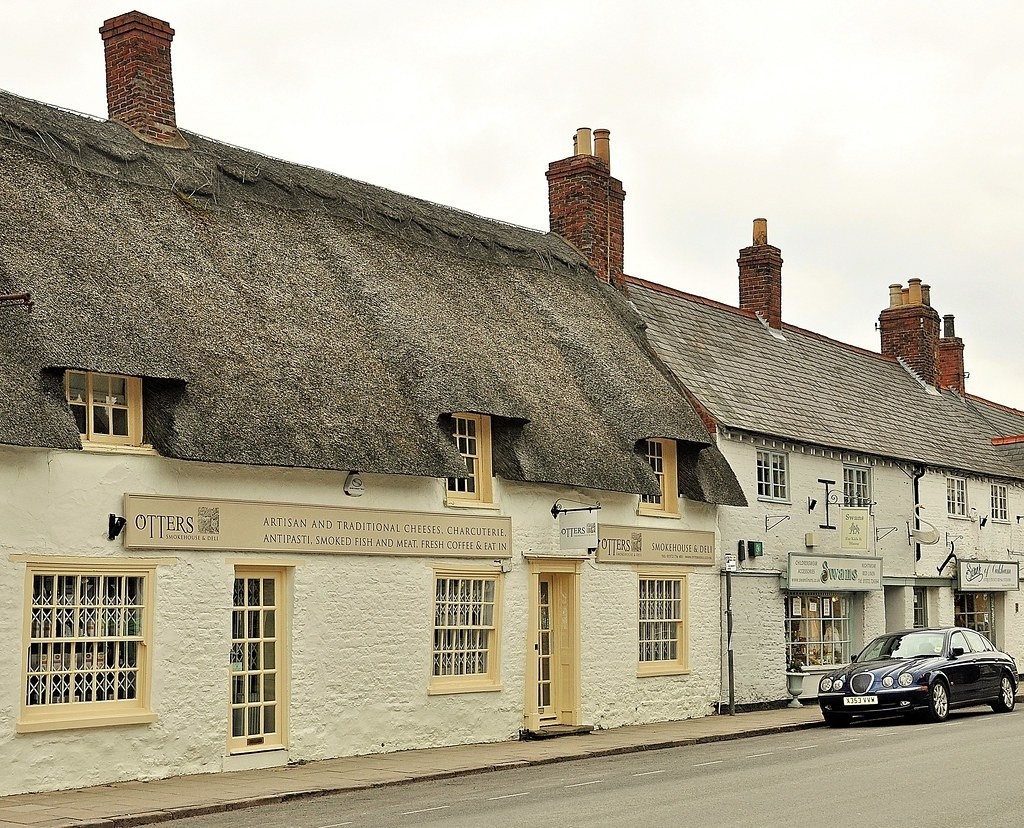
[783,672,810,708]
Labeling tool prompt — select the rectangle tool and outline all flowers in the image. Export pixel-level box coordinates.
[786,661,803,673]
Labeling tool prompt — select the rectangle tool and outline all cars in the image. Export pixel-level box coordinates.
[817,626,1019,728]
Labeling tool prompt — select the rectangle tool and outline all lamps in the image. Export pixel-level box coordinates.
[109,513,126,539]
[979,515,987,530]
